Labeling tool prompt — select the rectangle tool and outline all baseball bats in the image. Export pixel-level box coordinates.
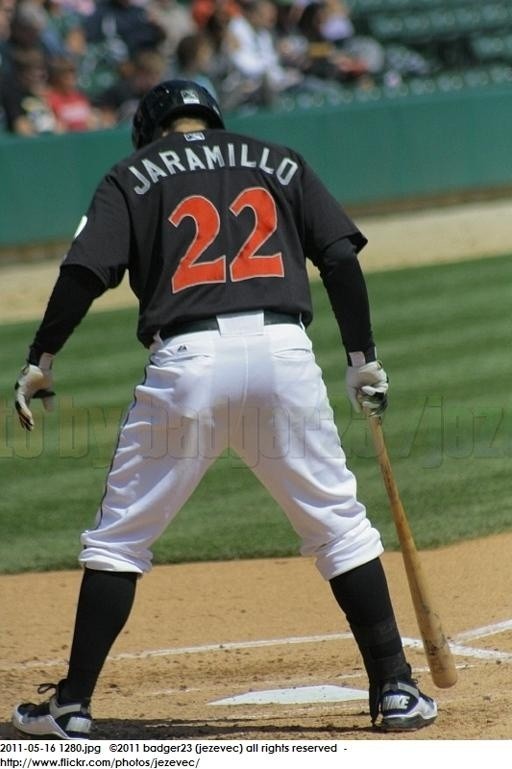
[357,384,459,688]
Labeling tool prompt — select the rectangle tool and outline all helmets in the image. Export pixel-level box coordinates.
[130,80,227,151]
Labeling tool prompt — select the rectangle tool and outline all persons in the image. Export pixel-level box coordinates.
[11,80,437,740]
[0,0,401,141]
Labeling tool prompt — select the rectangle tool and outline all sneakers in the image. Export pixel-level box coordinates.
[372,664,437,732]
[12,679,93,740]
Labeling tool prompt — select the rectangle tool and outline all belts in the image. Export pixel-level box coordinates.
[159,311,299,339]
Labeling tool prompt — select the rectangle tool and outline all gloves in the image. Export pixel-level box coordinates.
[346,347,389,418]
[13,350,55,432]
